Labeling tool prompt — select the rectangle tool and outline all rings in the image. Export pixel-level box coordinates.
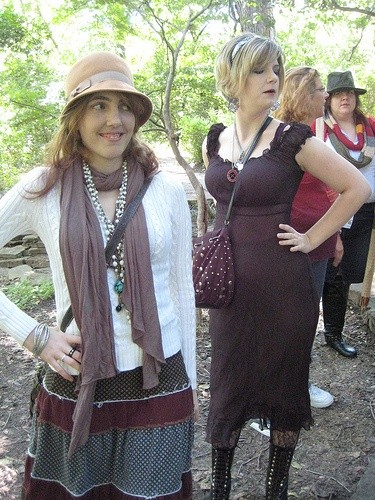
[61,354,67,363]
[68,348,77,356]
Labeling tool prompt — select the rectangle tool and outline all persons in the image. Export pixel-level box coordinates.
[312,72,375,359]
[0,52,199,500]
[205,36,372,500]
[273,66,344,311]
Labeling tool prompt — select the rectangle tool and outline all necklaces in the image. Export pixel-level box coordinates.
[82,160,129,310]
[226,117,267,182]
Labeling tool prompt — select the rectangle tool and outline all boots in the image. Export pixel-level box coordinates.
[266,439,296,500]
[211,444,237,500]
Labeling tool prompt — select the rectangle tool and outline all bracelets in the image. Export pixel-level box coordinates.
[31,321,50,358]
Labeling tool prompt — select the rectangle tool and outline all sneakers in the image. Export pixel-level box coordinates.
[308,381,334,408]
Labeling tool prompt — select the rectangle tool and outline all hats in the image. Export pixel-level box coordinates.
[326,71,367,95]
[59,51,154,133]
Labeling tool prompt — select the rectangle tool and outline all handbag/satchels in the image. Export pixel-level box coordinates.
[30,361,49,419]
[192,226,236,309]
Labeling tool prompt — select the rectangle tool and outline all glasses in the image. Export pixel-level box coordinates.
[316,87,325,92]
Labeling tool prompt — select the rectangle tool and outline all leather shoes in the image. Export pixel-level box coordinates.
[325,335,357,357]
